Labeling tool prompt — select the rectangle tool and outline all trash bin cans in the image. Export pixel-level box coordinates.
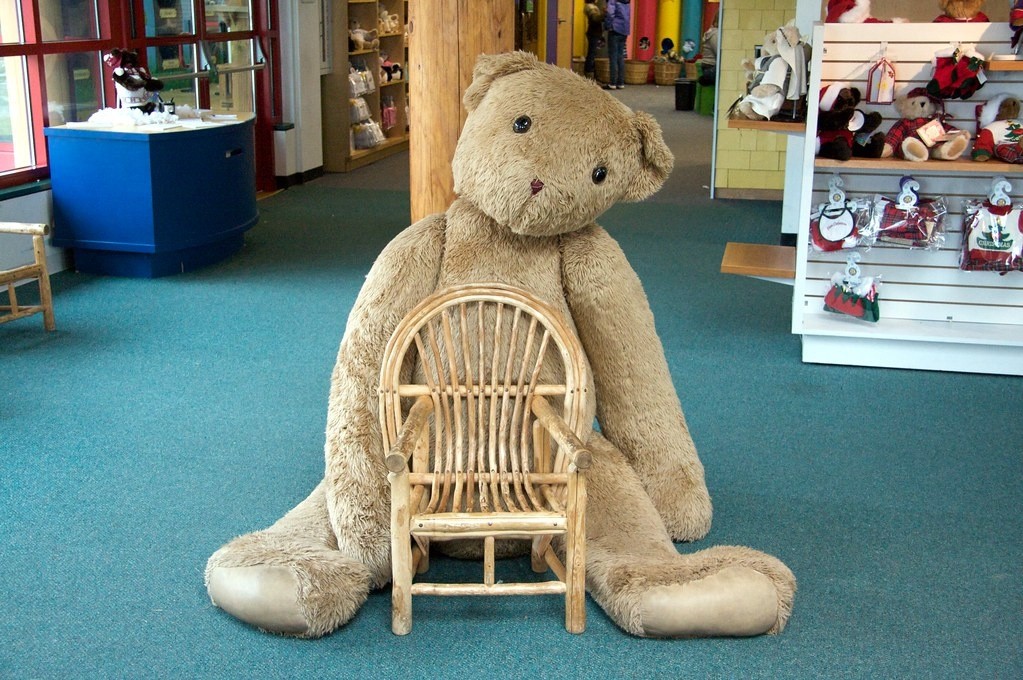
[674,77,697,112]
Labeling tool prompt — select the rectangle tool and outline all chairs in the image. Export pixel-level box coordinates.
[377,281,593,634]
[693,58,715,116]
[0,220,57,332]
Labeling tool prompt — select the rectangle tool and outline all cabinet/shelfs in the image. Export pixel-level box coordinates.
[719,110,807,287]
[321,0,408,173]
[791,43,1023,176]
[42,107,261,277]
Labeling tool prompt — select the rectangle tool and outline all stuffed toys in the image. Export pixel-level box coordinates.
[931,0,991,22]
[347,16,380,50]
[825,0,908,22]
[816,84,885,161]
[103,46,164,113]
[732,18,812,120]
[972,92,1023,164]
[204,49,798,639]
[379,3,399,34]
[379,49,403,83]
[881,87,971,162]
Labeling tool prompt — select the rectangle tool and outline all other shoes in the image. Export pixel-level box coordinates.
[603,84,616,89]
[617,84,625,89]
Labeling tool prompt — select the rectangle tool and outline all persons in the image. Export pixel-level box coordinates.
[700,10,719,86]
[660,38,685,64]
[583,0,607,84]
[602,0,631,89]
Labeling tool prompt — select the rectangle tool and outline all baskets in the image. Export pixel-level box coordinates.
[686,63,698,79]
[654,61,682,85]
[593,58,610,83]
[623,59,649,84]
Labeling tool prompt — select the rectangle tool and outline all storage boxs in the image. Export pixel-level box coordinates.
[594,58,699,88]
